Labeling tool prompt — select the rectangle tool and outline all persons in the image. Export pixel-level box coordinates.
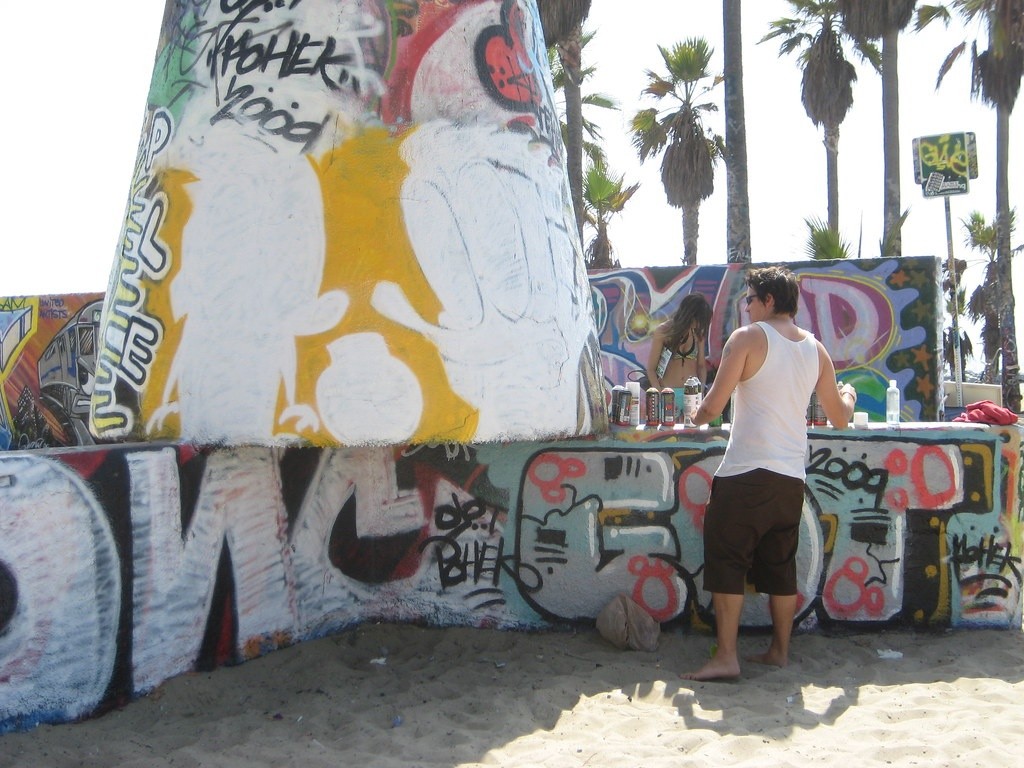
[648,293,713,423]
[680,266,858,681]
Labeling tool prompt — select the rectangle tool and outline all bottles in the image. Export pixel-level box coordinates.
[805,389,827,426]
[610,376,703,428]
[886,380,900,427]
[730,387,736,424]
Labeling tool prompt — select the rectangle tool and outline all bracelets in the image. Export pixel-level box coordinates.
[842,392,856,401]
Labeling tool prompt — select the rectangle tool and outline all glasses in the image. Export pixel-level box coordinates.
[746,294,759,305]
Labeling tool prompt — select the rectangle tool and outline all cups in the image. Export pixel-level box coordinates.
[854,411,868,426]
